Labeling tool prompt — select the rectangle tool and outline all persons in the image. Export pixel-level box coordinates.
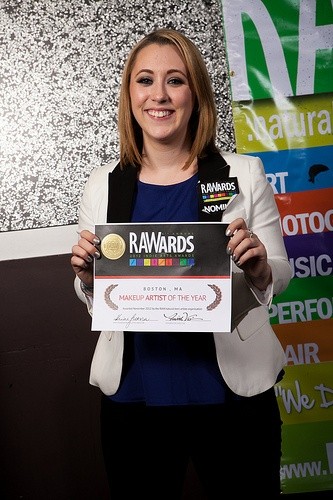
[69,27,294,500]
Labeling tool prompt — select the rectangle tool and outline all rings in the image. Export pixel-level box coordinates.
[248,229,254,239]
